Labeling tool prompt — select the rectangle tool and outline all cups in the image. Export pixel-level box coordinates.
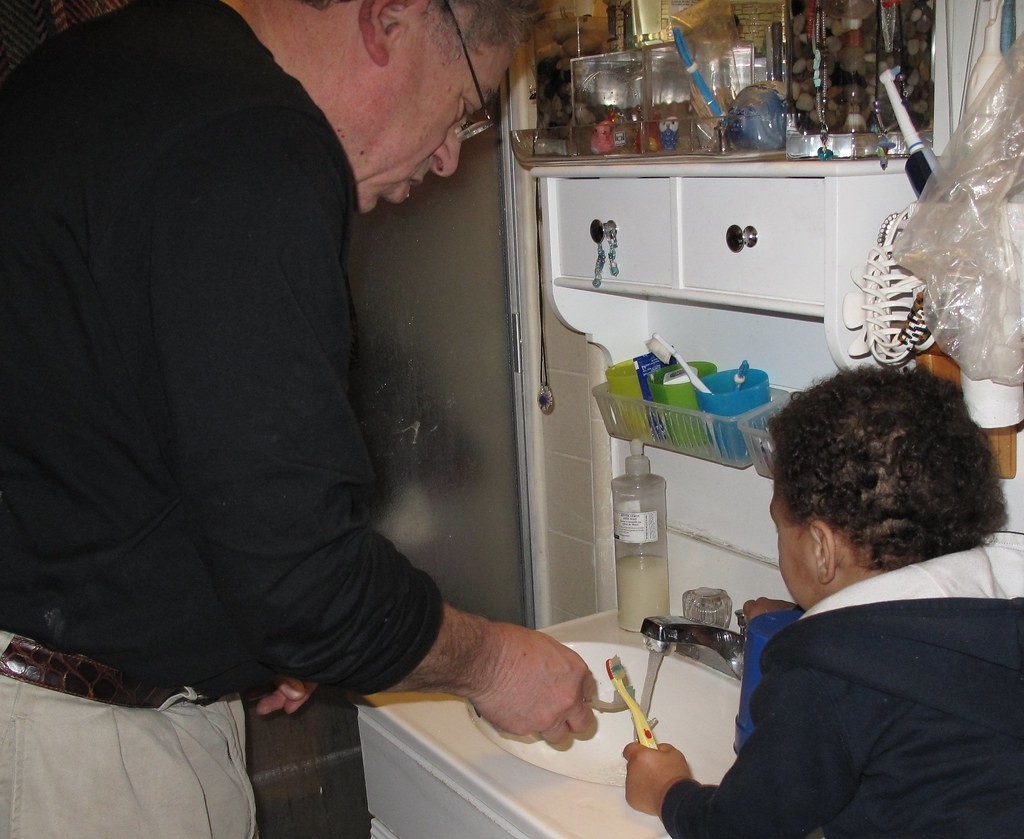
[694,369,771,460]
[605,358,650,435]
[647,361,717,447]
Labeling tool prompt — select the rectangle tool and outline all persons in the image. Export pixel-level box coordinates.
[0,0,601,839]
[624,363,1024,839]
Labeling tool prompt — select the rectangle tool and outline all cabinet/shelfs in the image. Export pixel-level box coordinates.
[529,168,918,389]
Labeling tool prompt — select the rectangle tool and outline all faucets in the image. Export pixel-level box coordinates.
[640,584,751,684]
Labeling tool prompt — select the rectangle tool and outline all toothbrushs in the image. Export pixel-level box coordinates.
[646,333,714,397]
[604,652,657,751]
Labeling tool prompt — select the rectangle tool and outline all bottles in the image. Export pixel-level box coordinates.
[611,438,670,633]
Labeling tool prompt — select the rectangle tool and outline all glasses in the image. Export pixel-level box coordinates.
[446,1,495,142]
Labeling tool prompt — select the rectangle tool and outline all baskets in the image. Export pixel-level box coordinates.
[592,383,790,469]
[738,395,800,479]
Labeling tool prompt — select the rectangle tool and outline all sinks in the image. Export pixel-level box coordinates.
[464,636,743,794]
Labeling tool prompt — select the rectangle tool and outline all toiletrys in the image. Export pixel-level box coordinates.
[608,439,672,632]
[633,345,676,441]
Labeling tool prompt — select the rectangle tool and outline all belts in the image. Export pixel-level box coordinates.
[1,639,219,708]
